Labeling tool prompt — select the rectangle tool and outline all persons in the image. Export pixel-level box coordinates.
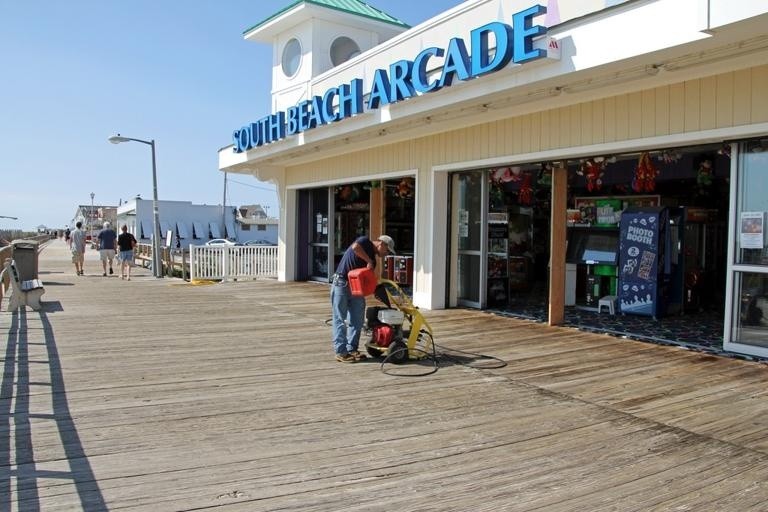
[117,224,137,281]
[69,222,85,276]
[64,228,71,250]
[329,234,396,363]
[96,221,117,276]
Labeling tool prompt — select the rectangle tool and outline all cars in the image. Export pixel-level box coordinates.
[243,239,278,253]
[195,238,242,255]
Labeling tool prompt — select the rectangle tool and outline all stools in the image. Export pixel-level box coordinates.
[598,295,620,315]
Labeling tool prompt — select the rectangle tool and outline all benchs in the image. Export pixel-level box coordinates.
[2,257,46,312]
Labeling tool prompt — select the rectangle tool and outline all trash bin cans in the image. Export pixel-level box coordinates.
[12,239,39,282]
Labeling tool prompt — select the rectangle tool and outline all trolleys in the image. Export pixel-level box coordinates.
[738,288,763,325]
[364,278,433,364]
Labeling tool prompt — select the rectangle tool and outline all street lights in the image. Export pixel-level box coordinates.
[91,190,95,240]
[264,205,271,216]
[105,133,164,280]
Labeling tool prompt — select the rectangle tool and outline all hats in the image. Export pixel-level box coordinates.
[377,234,396,255]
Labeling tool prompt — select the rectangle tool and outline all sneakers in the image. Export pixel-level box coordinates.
[348,351,367,358]
[120,275,131,281]
[103,268,114,276]
[335,354,357,364]
[76,269,84,276]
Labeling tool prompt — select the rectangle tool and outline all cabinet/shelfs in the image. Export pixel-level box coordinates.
[392,255,413,285]
[473,221,509,304]
[382,255,393,281]
